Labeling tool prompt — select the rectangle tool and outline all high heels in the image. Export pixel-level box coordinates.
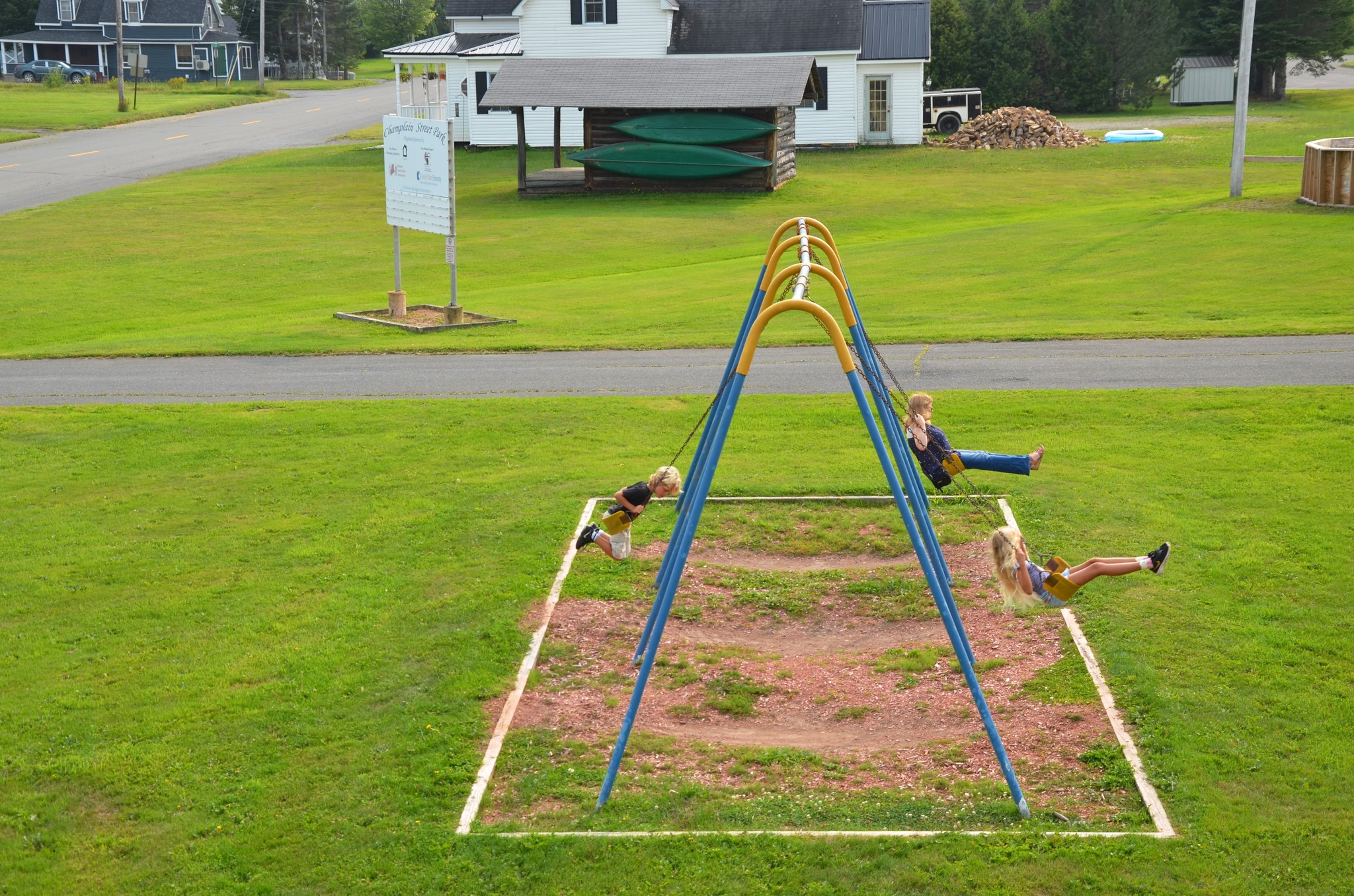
[1030,444,1044,470]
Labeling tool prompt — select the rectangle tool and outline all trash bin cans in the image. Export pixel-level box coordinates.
[95,71,103,83]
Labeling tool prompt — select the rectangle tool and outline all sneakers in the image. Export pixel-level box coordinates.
[589,521,598,532]
[576,524,594,550]
[1149,544,1171,576]
[1148,542,1169,558]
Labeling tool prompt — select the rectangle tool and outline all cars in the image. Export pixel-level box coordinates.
[14,59,97,84]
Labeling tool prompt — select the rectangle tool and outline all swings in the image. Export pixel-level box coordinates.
[798,274,1081,602]
[795,241,966,476]
[602,273,801,536]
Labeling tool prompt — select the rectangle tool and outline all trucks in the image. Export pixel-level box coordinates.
[923,87,982,135]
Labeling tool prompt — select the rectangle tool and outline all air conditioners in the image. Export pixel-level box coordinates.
[195,60,210,70]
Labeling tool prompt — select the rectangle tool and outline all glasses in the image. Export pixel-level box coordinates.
[926,407,933,411]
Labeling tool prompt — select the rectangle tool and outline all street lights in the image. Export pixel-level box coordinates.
[926,75,932,91]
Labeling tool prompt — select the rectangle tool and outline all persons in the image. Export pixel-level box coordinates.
[576,466,681,562]
[992,527,1172,616]
[902,392,1045,489]
